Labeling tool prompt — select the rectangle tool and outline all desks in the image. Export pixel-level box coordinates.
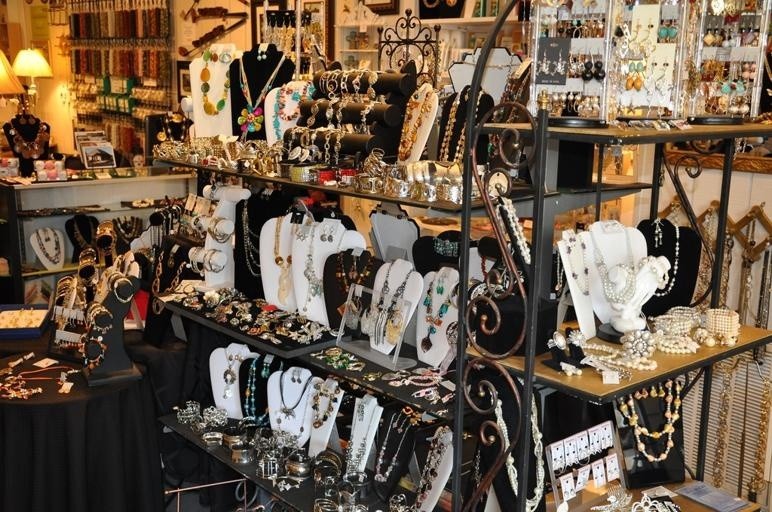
[0,329,169,511]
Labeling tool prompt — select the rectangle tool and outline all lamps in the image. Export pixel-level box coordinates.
[11,45,58,118]
[0,46,25,111]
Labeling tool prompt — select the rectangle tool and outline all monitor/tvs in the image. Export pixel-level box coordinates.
[145,114,164,166]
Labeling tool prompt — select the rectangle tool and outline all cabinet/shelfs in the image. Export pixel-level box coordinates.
[144,114,547,512]
[478,122,772,511]
[2,171,198,309]
